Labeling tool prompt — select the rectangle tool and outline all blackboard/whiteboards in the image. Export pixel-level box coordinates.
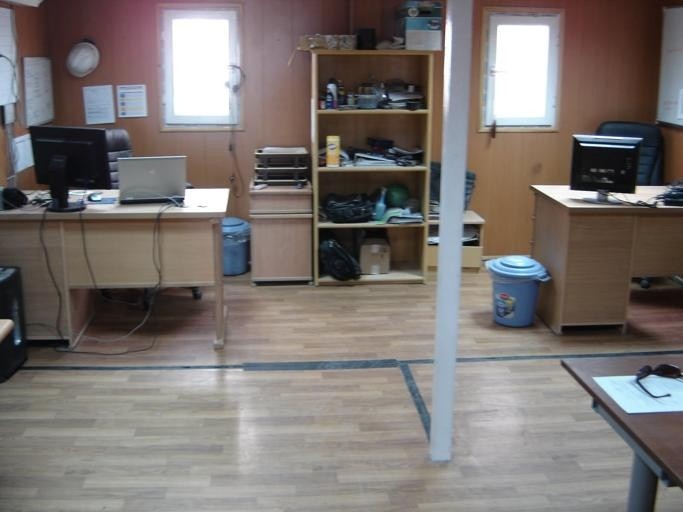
[21,54,60,129]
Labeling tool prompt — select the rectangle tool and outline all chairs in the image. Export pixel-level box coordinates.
[102,129,202,311]
[596,120,664,288]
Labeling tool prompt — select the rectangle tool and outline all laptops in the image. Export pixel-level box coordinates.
[118,155,187,204]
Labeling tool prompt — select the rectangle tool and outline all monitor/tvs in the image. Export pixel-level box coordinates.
[28,125,111,213]
[570,132,643,206]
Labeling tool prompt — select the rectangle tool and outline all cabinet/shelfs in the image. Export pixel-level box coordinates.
[311,50,434,285]
[248,177,314,285]
[429,210,485,271]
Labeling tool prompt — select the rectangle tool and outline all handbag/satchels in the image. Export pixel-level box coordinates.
[324,192,374,223]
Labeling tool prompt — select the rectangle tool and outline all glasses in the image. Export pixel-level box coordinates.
[636,365,683,398]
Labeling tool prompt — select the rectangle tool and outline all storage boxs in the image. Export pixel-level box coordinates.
[397,1,443,51]
[356,229,390,274]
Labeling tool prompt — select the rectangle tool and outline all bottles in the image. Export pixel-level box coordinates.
[374,187,387,220]
[325,135,341,168]
[320,77,345,109]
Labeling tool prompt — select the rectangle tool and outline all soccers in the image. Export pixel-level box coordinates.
[385,186,408,209]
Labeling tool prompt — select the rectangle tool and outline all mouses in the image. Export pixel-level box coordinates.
[88,193,102,201]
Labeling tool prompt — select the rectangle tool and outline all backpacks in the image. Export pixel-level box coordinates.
[319,239,362,281]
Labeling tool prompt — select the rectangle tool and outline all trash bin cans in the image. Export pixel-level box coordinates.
[488,254,552,327]
[221,216,250,277]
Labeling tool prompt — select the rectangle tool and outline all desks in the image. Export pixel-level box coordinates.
[561,351,683,512]
[0,188,231,351]
[528,183,683,336]
